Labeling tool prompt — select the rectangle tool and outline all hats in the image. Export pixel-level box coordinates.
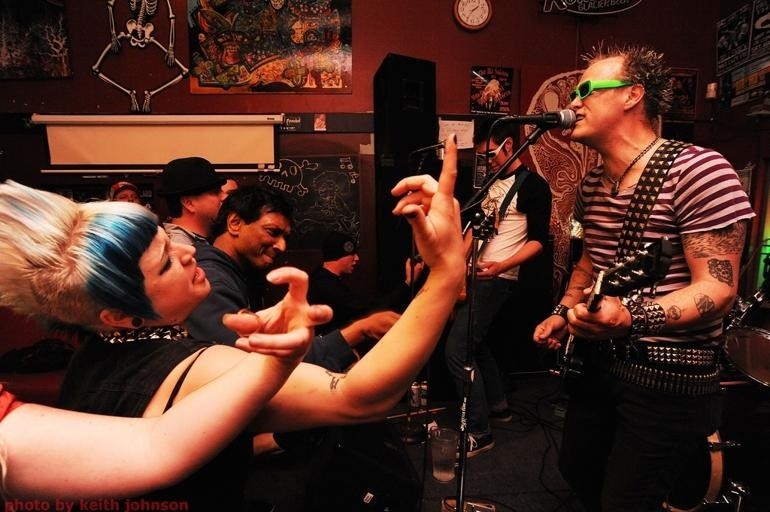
[155,157,227,194]
[110,181,138,200]
[322,232,358,262]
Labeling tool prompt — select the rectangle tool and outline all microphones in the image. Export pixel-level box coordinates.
[499,109,577,129]
[408,139,446,156]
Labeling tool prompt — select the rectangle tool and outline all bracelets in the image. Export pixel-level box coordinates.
[621,293,668,341]
[551,302,571,324]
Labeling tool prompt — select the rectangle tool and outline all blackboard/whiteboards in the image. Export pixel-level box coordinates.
[219,154,362,253]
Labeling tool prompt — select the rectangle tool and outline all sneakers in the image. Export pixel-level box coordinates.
[455,434,494,457]
[491,408,512,422]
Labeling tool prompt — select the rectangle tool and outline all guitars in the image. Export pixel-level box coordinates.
[550,236,682,386]
[436,203,506,317]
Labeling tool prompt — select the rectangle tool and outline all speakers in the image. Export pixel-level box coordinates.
[372,52,437,158]
[374,157,471,305]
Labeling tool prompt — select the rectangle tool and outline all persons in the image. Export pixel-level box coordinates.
[443,115,552,463]
[0,131,481,512]
[530,45,759,512]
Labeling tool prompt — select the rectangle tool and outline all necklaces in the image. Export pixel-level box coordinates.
[600,135,661,198]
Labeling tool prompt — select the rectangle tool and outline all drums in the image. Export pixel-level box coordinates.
[725,291,769,393]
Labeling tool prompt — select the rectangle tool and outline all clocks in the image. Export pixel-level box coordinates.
[454,0,493,30]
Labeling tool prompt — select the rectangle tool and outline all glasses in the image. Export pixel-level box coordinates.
[568,79,634,101]
[476,138,510,160]
